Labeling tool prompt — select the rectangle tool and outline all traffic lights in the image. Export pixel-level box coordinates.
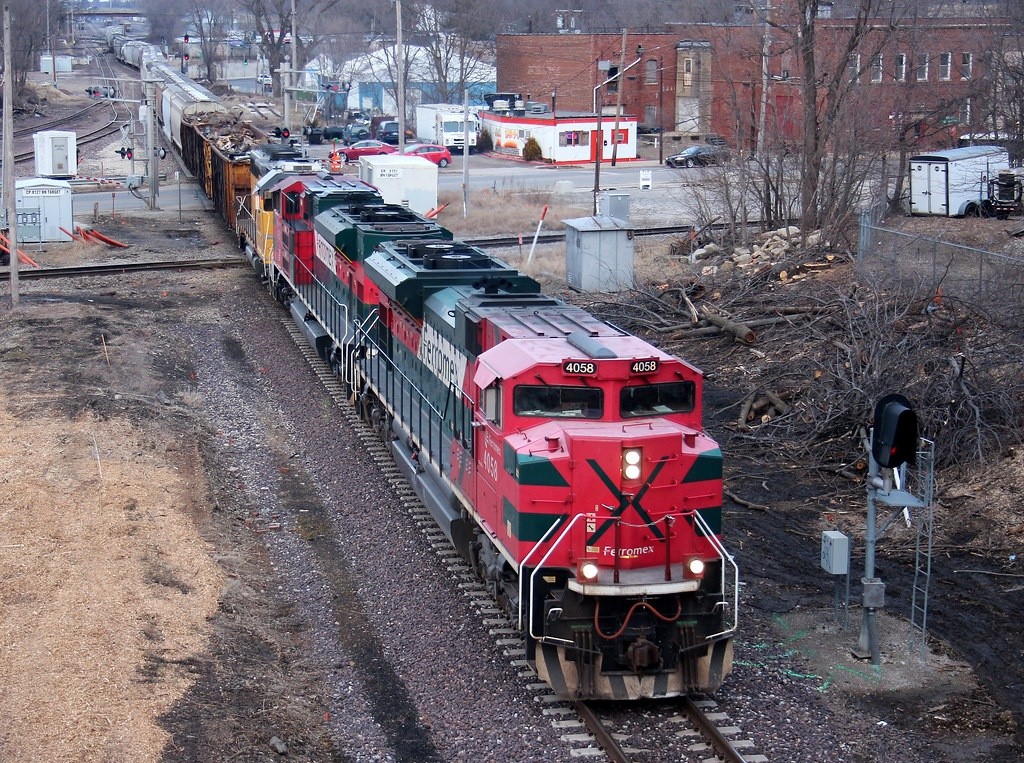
[184,35,189,43]
[84,86,100,96]
[877,403,918,470]
[115,147,132,160]
[185,54,188,60]
[282,128,289,138]
[270,127,281,138]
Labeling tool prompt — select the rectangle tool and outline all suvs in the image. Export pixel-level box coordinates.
[375,120,406,144]
[342,122,371,146]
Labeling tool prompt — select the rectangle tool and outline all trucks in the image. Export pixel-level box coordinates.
[415,103,480,155]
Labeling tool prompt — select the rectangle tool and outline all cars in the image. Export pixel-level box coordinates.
[665,144,731,169]
[389,144,452,168]
[328,139,398,162]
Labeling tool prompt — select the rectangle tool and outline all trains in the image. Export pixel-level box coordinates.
[103,15,737,704]
